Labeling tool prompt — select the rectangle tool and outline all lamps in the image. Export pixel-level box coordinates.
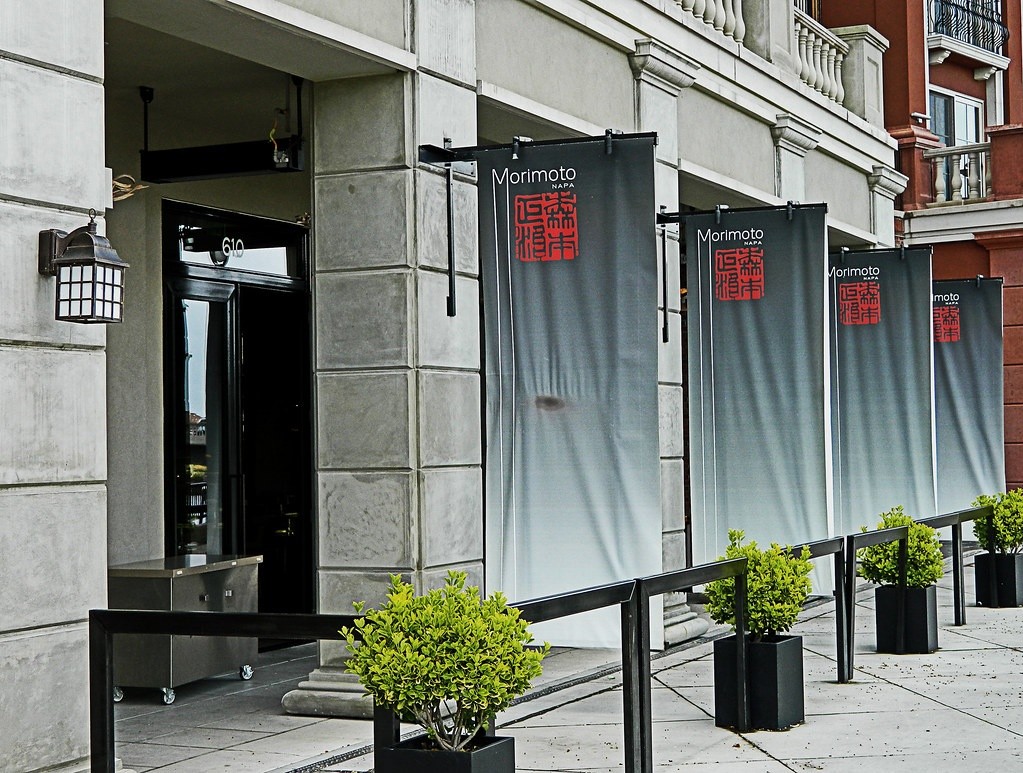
[39,208,130,324]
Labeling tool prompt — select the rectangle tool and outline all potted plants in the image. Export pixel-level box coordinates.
[339,569,554,773]
[971,486,1023,607]
[699,529,815,727]
[857,504,946,655]
